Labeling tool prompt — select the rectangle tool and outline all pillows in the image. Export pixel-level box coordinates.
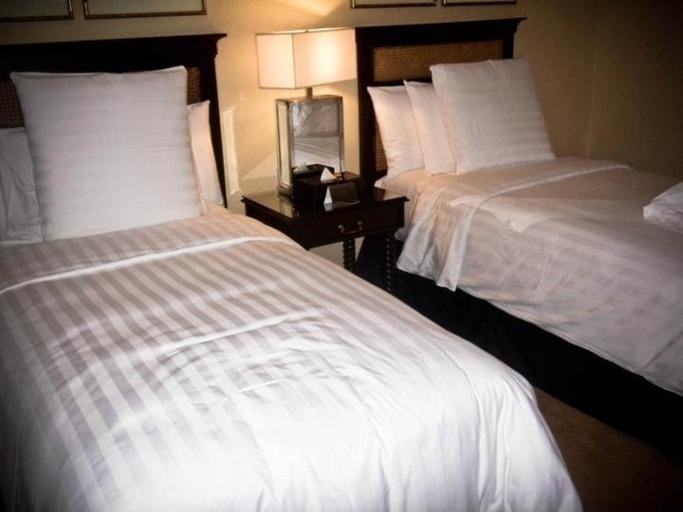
[366,51,556,183]
[10,67,206,243]
[0,99,224,242]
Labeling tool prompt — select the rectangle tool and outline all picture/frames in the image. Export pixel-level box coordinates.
[83,0,207,19]
[0,0,74,23]
[441,0,516,7]
[350,0,437,9]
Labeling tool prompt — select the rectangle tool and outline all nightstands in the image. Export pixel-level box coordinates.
[241,187,411,294]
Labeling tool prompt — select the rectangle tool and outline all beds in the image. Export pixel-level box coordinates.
[0,31,584,512]
[354,14,683,465]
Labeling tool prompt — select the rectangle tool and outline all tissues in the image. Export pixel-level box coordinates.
[293,167,362,213]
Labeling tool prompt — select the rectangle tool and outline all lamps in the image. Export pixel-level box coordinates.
[253,25,364,198]
